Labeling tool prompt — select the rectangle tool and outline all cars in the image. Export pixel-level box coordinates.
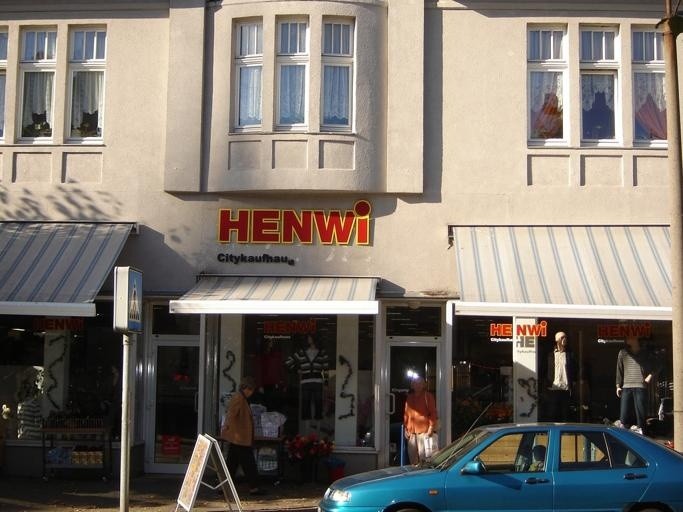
[319,422,683,511]
[355,420,402,468]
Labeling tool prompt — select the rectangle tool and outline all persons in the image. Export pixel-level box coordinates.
[283,331,328,435]
[614,336,663,434]
[216,375,267,496]
[401,375,437,465]
[14,360,122,444]
[542,331,577,422]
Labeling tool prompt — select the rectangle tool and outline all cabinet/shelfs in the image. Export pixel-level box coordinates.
[42,417,113,482]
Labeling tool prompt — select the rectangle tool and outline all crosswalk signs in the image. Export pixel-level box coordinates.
[128,267,143,337]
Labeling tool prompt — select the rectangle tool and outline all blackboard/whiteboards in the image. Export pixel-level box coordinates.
[176,433,213,512]
[205,433,242,504]
